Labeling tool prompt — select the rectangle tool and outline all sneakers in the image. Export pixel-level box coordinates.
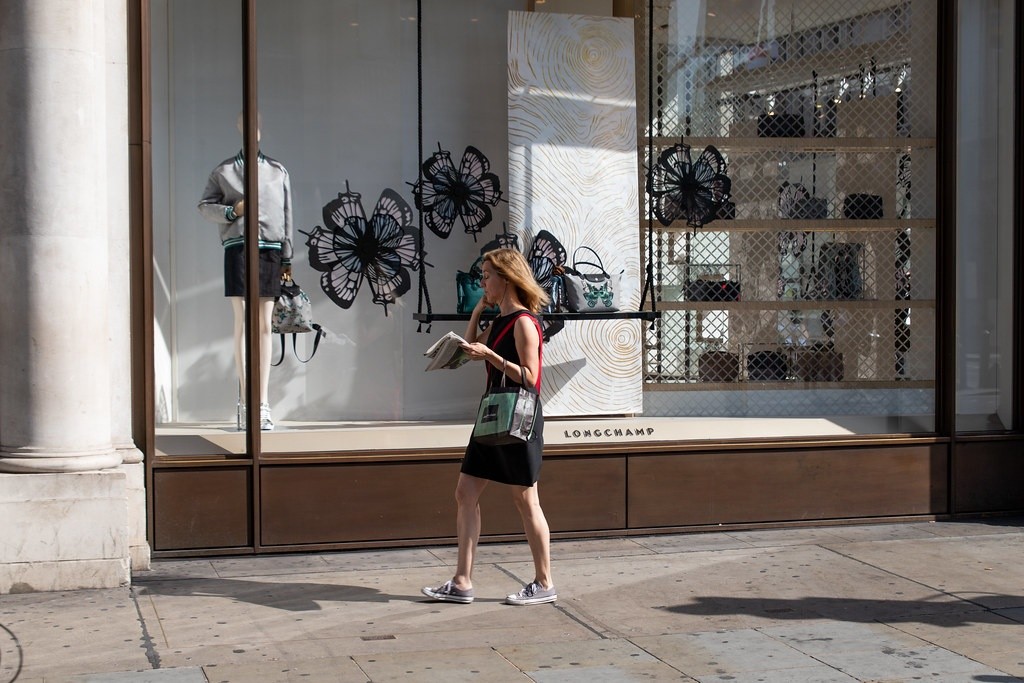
[505,579,557,605]
[421,576,475,603]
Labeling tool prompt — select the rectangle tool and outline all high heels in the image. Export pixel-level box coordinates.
[260,402,274,430]
[237,401,246,430]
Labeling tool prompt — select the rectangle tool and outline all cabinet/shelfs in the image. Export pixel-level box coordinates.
[644,135,936,388]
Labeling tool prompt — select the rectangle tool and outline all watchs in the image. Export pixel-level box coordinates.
[232,211,240,218]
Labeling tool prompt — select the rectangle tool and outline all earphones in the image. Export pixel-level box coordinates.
[505,278,509,281]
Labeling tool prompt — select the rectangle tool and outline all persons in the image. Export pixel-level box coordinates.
[420,248,559,605]
[197,111,294,432]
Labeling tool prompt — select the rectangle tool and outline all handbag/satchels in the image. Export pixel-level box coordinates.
[757,114,806,137]
[537,266,564,313]
[843,194,884,219]
[707,201,735,220]
[698,351,740,381]
[456,258,501,313]
[796,353,844,382]
[562,246,624,313]
[747,351,788,380]
[689,279,741,302]
[795,198,827,219]
[472,359,540,446]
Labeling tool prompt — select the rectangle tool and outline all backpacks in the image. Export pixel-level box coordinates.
[271,275,326,367]
[818,242,862,299]
